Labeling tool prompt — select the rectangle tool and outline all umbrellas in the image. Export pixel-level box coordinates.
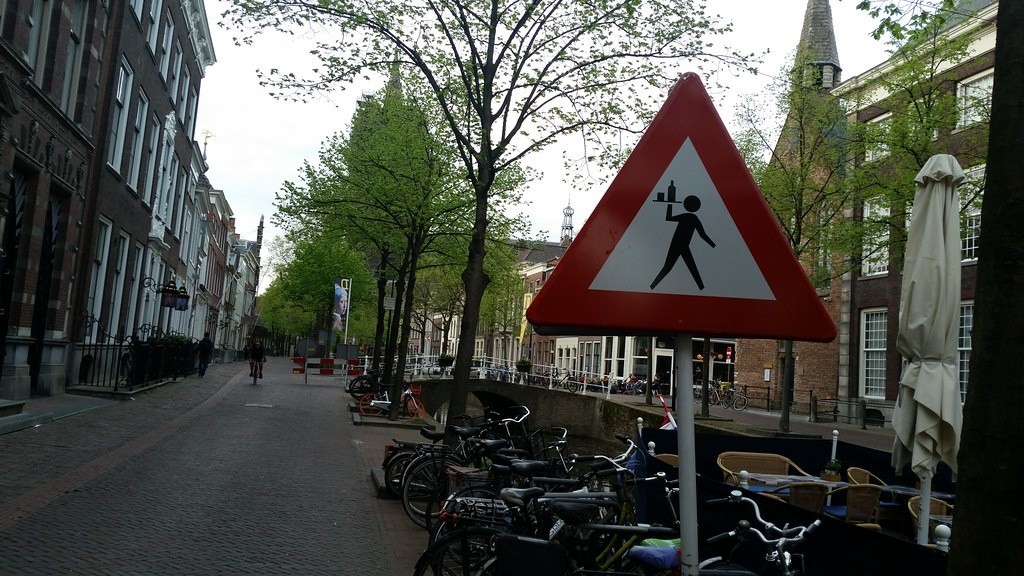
[882,151,961,551]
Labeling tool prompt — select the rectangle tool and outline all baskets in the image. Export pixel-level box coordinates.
[411,384,422,395]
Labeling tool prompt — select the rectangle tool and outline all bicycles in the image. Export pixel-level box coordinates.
[248,356,266,385]
[428,359,456,380]
[348,362,424,420]
[488,363,748,410]
[381,403,822,576]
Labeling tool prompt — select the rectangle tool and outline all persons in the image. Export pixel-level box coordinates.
[195,331,215,377]
[246,341,266,380]
[334,283,347,332]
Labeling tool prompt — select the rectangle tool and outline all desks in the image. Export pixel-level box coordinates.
[743,471,852,504]
[881,483,953,498]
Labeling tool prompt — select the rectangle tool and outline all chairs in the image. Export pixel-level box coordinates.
[650,379,664,395]
[623,380,642,394]
[657,452,955,530]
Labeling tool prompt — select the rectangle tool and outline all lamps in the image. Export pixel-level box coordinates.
[142,277,191,311]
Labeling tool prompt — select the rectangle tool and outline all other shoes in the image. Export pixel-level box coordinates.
[259,373,263,379]
[249,372,253,377]
